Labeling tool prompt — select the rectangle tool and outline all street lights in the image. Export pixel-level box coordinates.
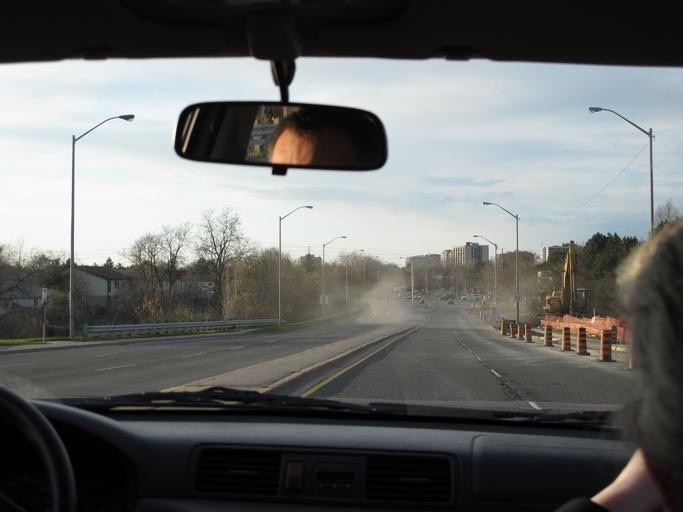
[481,199,521,341]
[276,204,314,326]
[344,249,381,307]
[585,104,655,241]
[398,256,414,304]
[64,112,136,341]
[471,234,498,308]
[320,235,347,318]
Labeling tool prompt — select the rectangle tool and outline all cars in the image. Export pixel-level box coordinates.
[461,293,480,301]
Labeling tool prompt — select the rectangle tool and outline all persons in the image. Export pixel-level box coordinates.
[559,213,682,512]
[267,105,378,172]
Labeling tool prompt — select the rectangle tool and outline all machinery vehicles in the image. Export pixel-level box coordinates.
[542,241,593,317]
